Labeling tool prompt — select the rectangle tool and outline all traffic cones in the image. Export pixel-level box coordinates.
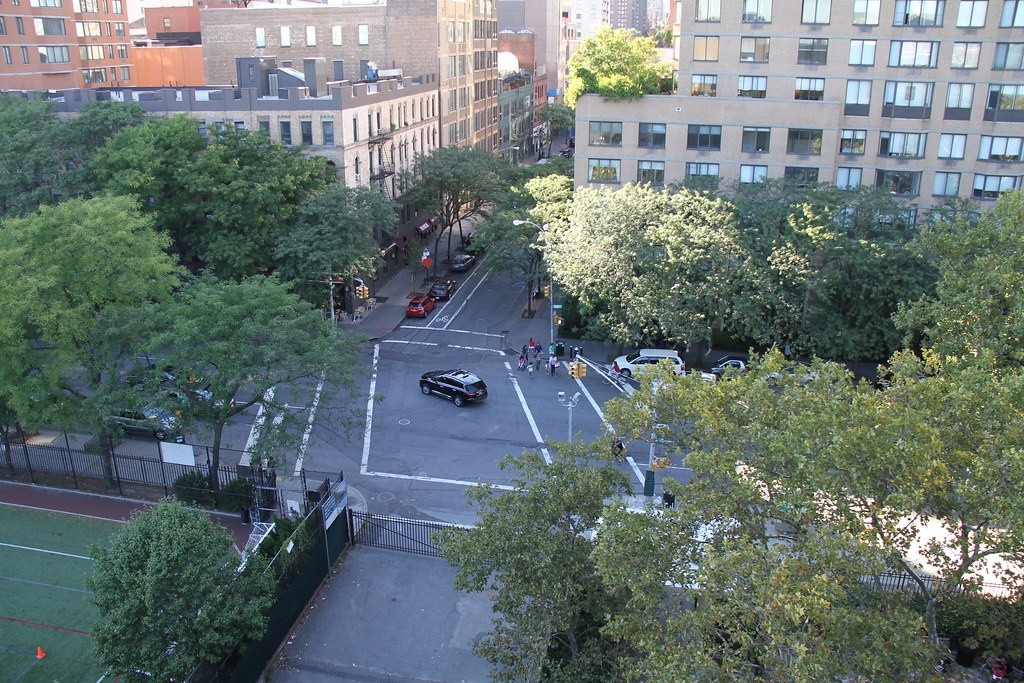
[35,646,46,659]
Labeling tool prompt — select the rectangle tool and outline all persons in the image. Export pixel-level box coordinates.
[519,338,557,376]
[612,439,623,466]
[612,361,620,383]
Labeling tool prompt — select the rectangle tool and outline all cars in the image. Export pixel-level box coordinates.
[430,280,456,301]
[99,364,237,441]
[686,355,818,387]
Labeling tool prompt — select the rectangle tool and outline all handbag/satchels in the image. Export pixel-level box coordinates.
[555,362,560,368]
[534,354,536,358]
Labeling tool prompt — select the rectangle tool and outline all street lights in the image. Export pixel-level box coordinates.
[558,391,581,442]
[513,219,554,344]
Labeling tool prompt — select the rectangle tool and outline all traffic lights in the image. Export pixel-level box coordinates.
[651,462,657,472]
[364,287,369,300]
[185,367,194,383]
[356,286,364,299]
[579,362,587,378]
[568,361,576,376]
[571,365,578,379]
[544,285,550,298]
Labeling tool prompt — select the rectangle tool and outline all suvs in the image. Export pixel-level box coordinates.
[612,349,686,380]
[418,368,489,407]
[451,255,476,272]
[405,296,437,319]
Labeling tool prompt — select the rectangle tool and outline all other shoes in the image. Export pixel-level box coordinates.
[536,368,541,371]
[551,374,553,375]
[554,372,555,374]
